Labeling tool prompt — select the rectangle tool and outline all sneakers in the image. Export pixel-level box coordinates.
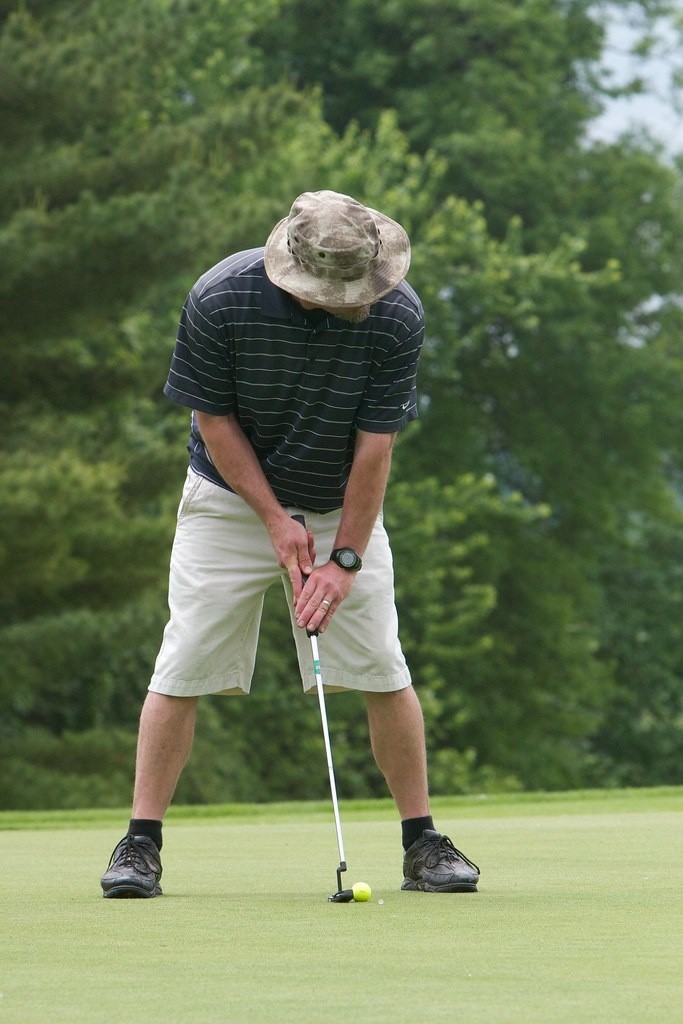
[401,829,480,892]
[100,834,163,898]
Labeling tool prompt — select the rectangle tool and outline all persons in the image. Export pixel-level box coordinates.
[100,189,480,896]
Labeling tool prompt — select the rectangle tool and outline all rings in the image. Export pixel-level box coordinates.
[322,600,330,607]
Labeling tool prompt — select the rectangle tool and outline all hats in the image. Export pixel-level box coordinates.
[264,189,411,307]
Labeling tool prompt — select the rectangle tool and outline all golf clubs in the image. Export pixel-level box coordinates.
[290,513,353,901]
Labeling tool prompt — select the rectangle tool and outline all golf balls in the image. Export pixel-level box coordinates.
[353,881,372,901]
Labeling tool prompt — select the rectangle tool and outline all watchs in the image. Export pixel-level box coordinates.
[330,548,362,571]
